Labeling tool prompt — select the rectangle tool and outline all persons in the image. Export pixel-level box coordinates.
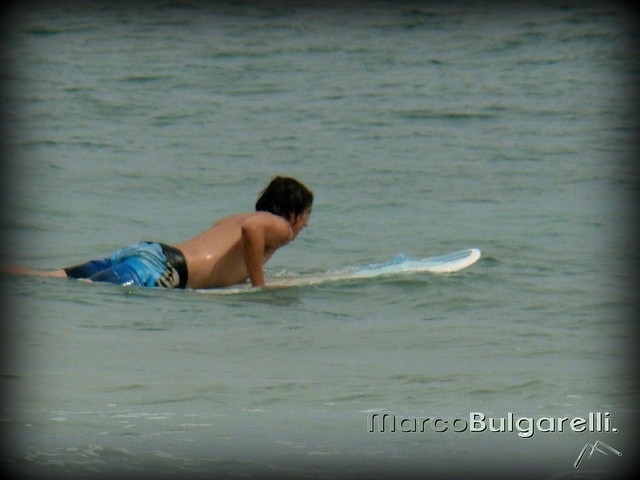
[0,177,314,290]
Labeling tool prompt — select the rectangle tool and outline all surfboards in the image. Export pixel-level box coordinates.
[186,247,482,295]
